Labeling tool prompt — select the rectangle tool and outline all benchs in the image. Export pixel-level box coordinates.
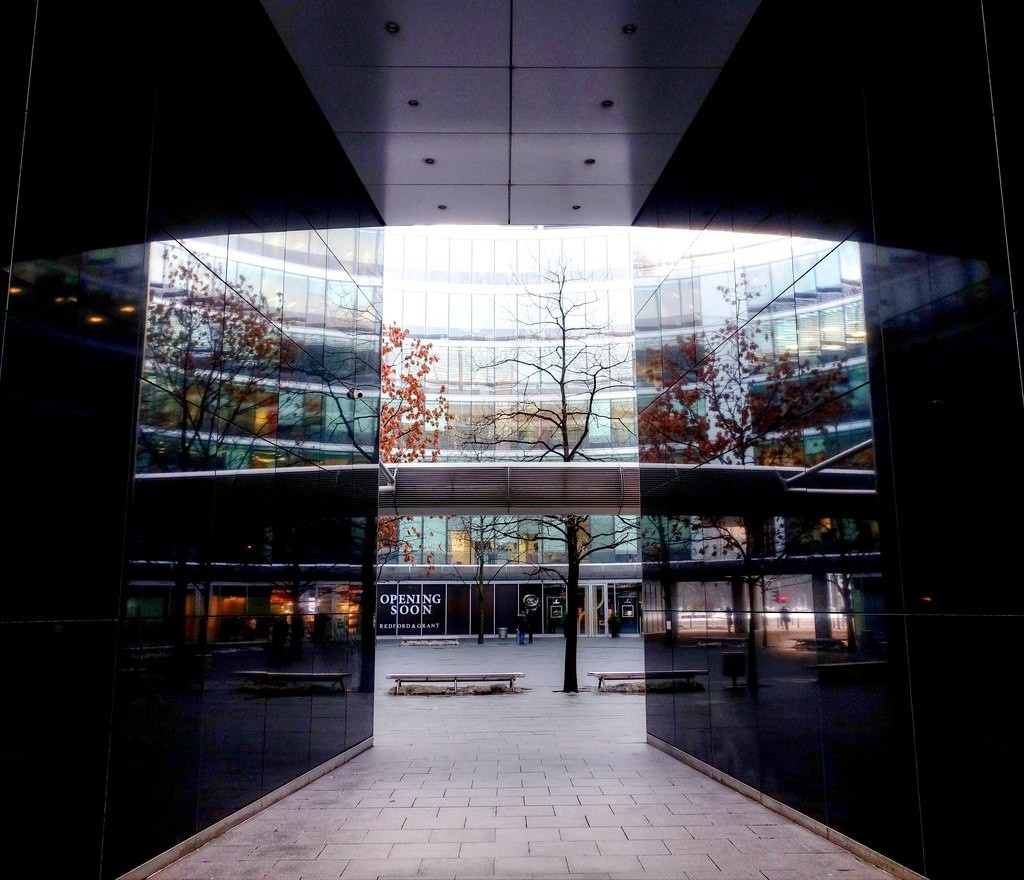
[690,636,749,647]
[646,670,708,689]
[235,671,352,696]
[587,671,645,694]
[129,645,172,659]
[798,660,886,682]
[787,638,847,651]
[386,672,526,696]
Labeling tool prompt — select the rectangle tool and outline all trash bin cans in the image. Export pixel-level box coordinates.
[515,629,525,645]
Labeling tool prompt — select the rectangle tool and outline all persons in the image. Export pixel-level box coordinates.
[290,613,304,661]
[780,607,791,631]
[272,616,288,652]
[726,607,733,633]
[517,611,527,644]
